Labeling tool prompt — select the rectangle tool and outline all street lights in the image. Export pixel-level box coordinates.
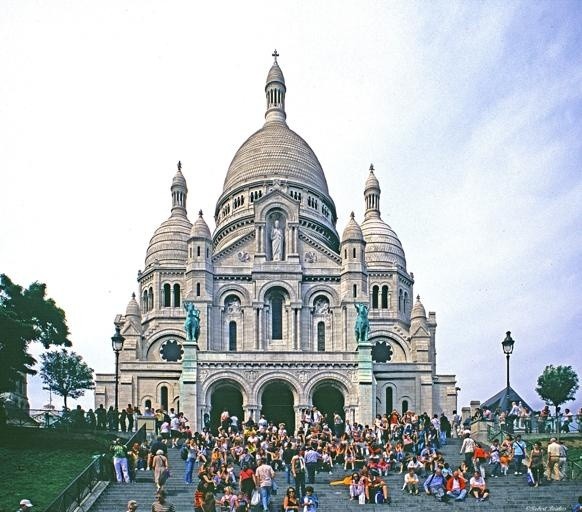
[110,325,126,409]
[501,331,515,414]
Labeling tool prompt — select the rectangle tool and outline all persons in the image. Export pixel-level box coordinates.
[77,402,582,512]
[16,499,33,512]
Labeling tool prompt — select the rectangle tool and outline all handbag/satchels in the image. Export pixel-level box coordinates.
[375,491,384,504]
[527,472,535,486]
[484,452,495,465]
[251,489,261,507]
[453,490,459,494]
[522,450,525,459]
[158,470,173,486]
[427,484,430,489]
[359,493,365,504]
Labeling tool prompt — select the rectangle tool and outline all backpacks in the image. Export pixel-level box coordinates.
[180,447,188,460]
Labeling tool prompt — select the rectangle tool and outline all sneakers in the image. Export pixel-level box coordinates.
[185,481,193,484]
[135,467,150,471]
[408,493,419,496]
[439,496,485,502]
[491,471,522,478]
